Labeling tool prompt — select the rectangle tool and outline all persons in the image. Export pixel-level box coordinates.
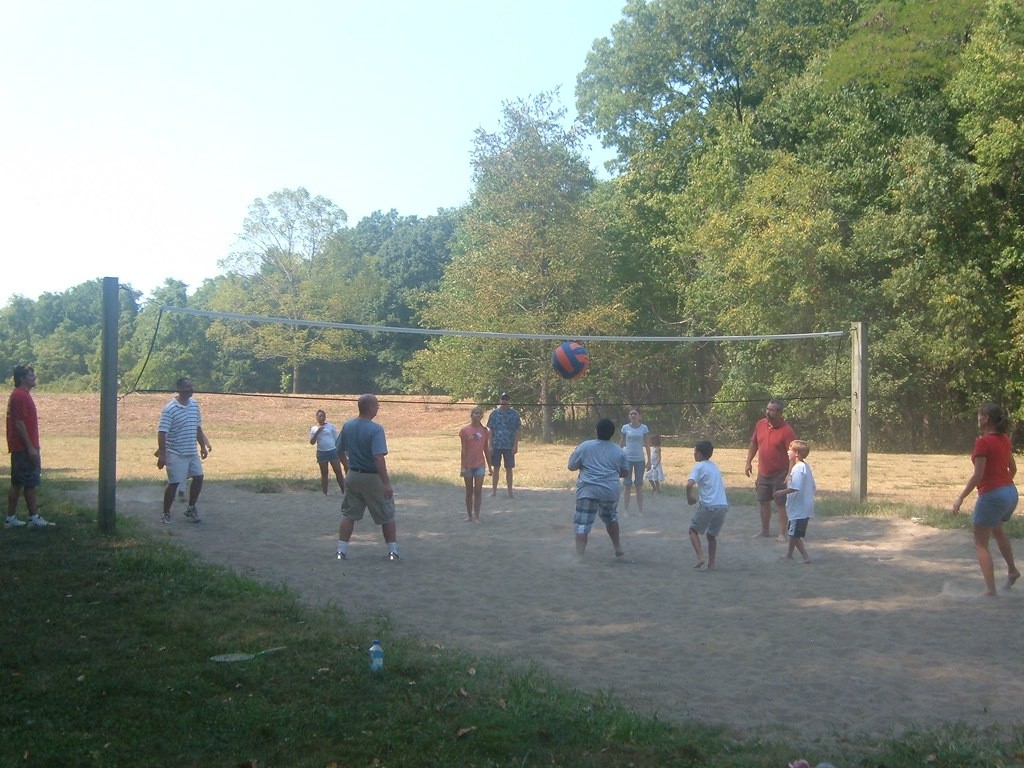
[568,418,629,561]
[646,436,665,491]
[157,379,212,528]
[952,403,1021,596]
[459,407,494,526]
[686,441,728,570]
[335,395,401,560]
[486,392,521,499]
[619,408,651,516]
[310,410,344,496]
[773,440,816,563]
[745,400,795,541]
[5,366,56,528]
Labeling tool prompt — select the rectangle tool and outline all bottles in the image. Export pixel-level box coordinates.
[369,640,383,672]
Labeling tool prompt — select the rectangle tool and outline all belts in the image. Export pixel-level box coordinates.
[350,468,376,474]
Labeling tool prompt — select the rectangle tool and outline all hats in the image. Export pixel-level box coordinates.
[500,393,508,397]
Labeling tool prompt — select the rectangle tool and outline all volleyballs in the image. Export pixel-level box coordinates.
[551,341,589,381]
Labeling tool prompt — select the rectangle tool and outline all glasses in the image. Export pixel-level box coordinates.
[502,398,509,401]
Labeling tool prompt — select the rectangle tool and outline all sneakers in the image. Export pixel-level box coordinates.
[184,507,200,522]
[4,519,26,527]
[160,512,172,525]
[28,517,55,527]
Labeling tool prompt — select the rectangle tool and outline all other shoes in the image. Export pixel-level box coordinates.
[335,551,345,560]
[389,553,399,560]
